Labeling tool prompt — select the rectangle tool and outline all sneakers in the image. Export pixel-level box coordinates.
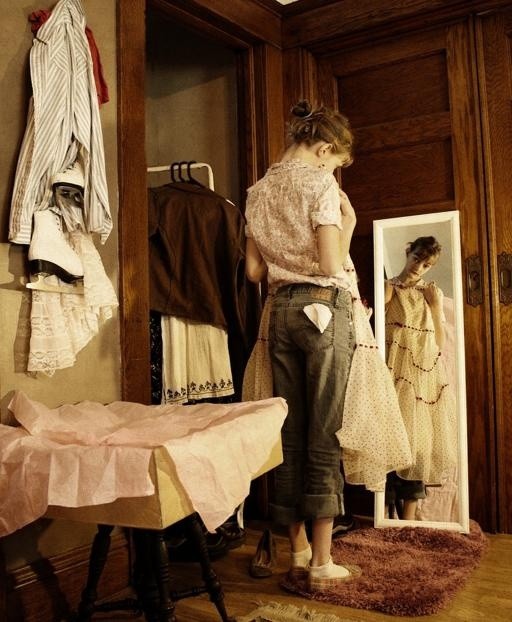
[289,566,311,582]
[308,563,361,592]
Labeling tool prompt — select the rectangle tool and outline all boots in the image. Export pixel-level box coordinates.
[30,211,85,282]
[50,158,84,202]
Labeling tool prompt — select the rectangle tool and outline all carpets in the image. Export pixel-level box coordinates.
[234,600,344,622]
[282,518,486,616]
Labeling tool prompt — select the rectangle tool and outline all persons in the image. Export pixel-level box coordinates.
[242,97,365,593]
[382,235,449,523]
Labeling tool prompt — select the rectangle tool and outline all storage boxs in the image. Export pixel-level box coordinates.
[43,430,286,533]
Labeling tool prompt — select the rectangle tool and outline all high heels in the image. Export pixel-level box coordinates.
[250,530,277,580]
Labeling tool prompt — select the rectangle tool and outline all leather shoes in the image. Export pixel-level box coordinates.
[202,532,228,560]
[217,523,246,546]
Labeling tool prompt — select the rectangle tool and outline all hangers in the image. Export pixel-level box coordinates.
[188,161,205,186]
[179,162,187,183]
[170,162,178,184]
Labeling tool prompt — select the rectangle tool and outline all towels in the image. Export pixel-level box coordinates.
[158,314,235,407]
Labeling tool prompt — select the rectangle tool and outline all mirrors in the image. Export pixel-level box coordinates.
[373,211,472,534]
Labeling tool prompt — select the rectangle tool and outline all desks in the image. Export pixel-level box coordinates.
[75,512,231,621]
